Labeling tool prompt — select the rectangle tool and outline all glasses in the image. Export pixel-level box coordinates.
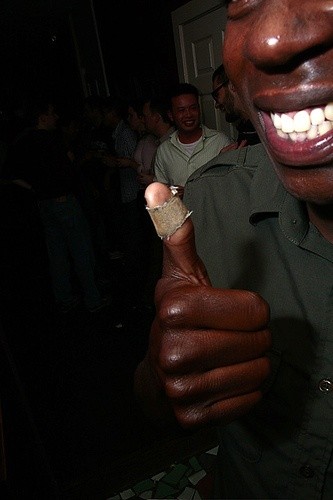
[211,80,229,100]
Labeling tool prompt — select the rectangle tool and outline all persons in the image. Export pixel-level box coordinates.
[134,0,333,500]
[0,64,262,314]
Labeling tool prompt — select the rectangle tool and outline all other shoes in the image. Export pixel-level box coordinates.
[110,250,123,259]
[62,297,80,313]
[90,295,112,312]
